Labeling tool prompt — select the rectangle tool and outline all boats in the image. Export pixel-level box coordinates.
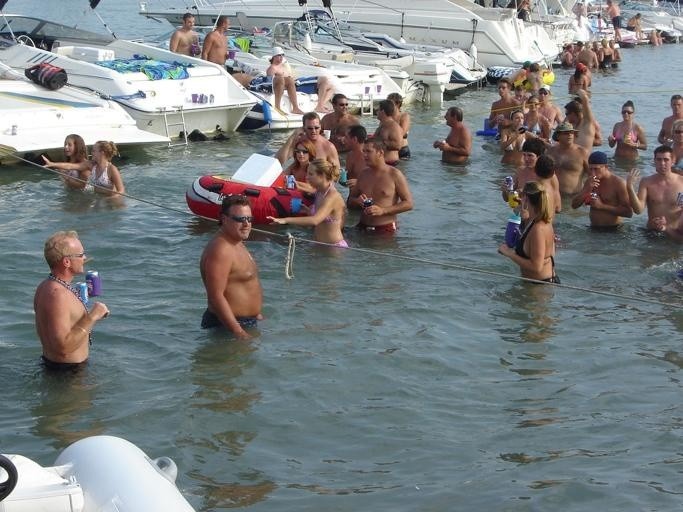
[0,430,202,512]
[0,58,173,164]
[0,0,267,143]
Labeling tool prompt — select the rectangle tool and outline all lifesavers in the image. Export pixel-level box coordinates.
[487,67,555,90]
[186,174,316,225]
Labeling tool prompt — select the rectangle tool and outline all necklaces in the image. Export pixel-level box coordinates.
[247,251,254,263]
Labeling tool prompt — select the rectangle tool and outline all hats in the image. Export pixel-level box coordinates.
[538,84,551,91]
[551,120,582,143]
[271,46,285,57]
[588,150,608,166]
[575,63,587,72]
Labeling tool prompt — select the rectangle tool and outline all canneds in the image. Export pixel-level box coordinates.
[339,169,346,183]
[440,139,446,151]
[509,190,519,207]
[76,282,88,304]
[365,198,374,207]
[286,175,295,189]
[505,177,513,190]
[86,270,100,296]
[624,134,631,144]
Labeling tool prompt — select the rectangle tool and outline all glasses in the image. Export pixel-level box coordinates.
[335,103,349,106]
[275,53,285,56]
[292,148,309,154]
[558,131,575,136]
[304,125,321,131]
[674,130,683,134]
[621,110,634,115]
[223,211,256,224]
[538,91,548,95]
[565,112,573,116]
[61,249,86,259]
[528,102,542,106]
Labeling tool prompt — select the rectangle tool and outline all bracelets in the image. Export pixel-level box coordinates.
[75,325,87,334]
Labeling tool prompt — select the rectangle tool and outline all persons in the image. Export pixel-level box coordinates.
[270,89,414,250]
[226,65,306,116]
[27,372,102,452]
[38,140,125,201]
[432,107,472,164]
[197,194,263,344]
[266,45,335,114]
[203,16,234,68]
[168,13,201,60]
[58,134,91,192]
[32,230,109,376]
[237,27,271,40]
